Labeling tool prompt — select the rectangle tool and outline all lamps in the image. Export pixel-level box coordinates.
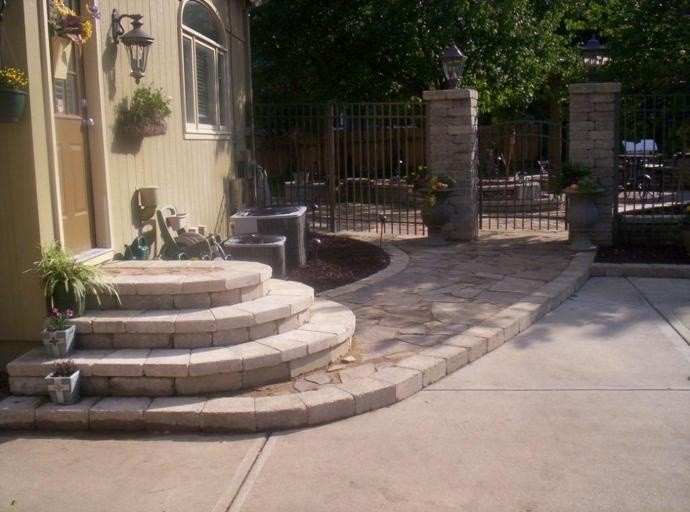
[111,9,155,83]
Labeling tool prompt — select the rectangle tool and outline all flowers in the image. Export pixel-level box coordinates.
[47,307,73,330]
[1,67,29,90]
[115,81,174,124]
[49,0,101,45]
[401,165,456,206]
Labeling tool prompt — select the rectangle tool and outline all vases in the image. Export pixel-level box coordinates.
[41,325,76,357]
[50,35,72,79]
[121,117,167,137]
[0,88,27,122]
[418,189,453,246]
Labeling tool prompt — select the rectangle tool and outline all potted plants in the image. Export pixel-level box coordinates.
[552,161,605,251]
[22,239,122,318]
[45,360,80,405]
